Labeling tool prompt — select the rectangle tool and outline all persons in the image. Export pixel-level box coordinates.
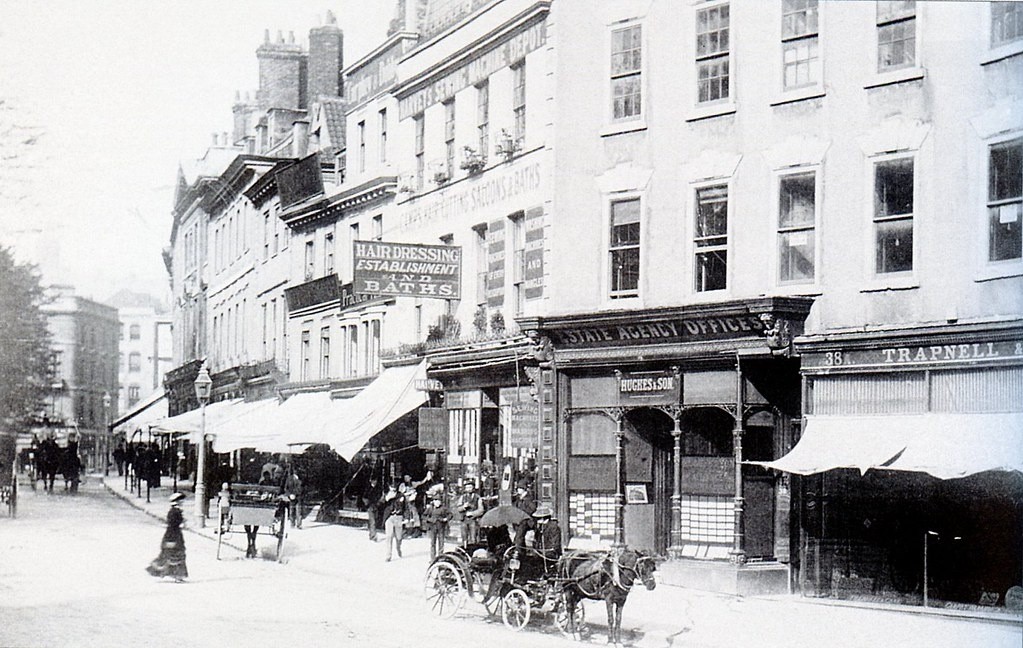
[19,412,78,456]
[144,493,189,583]
[204,455,306,528]
[361,458,561,594]
[113,438,198,493]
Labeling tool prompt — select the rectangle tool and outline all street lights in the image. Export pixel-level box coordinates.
[102,392,111,477]
[193,365,212,526]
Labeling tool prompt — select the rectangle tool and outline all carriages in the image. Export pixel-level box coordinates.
[28,437,80,493]
[421,529,656,647]
[212,482,290,563]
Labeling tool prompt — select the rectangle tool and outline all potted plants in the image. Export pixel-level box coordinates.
[432,128,517,186]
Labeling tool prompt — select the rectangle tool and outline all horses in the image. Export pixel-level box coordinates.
[62,440,80,496]
[552,544,656,648]
[36,437,61,493]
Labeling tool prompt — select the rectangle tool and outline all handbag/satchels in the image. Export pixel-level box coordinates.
[162,541,178,549]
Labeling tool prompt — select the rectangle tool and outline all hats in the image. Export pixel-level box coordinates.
[531,506,553,518]
[517,484,527,491]
[464,481,475,487]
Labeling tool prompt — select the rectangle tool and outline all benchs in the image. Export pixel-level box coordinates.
[229,484,280,507]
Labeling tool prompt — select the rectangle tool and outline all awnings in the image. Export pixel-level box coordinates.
[110,362,430,462]
[739,414,1023,481]
